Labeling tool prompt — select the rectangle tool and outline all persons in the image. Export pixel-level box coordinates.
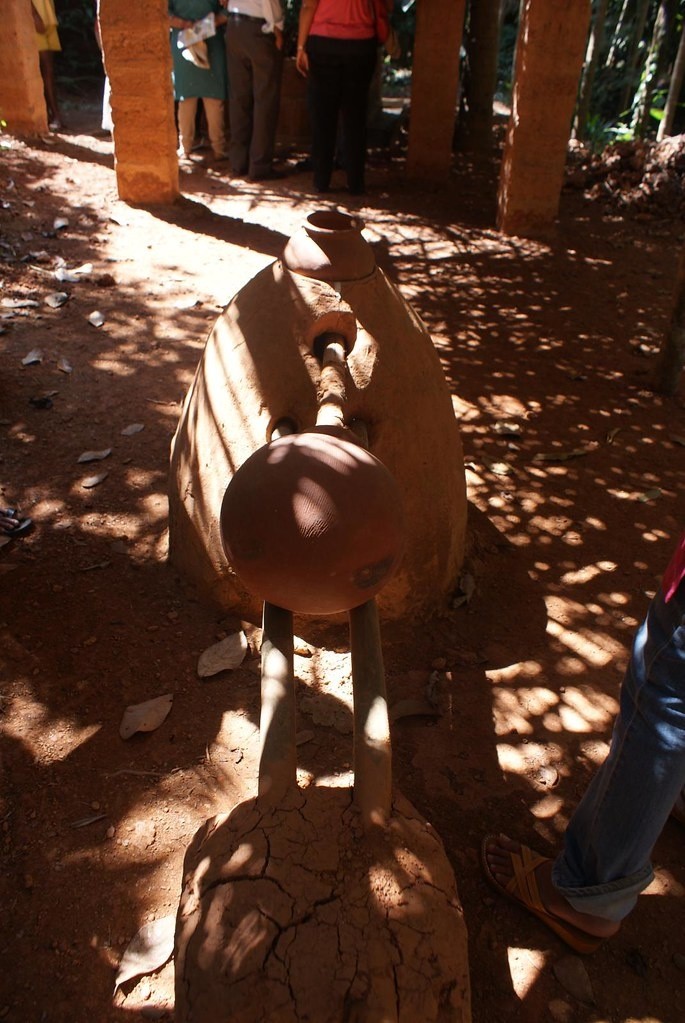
[99,0,401,195]
[31,0,68,130]
[481,534,685,954]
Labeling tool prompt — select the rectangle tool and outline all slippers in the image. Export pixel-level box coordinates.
[481,833,605,958]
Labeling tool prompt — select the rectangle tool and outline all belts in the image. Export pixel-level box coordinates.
[227,13,266,24]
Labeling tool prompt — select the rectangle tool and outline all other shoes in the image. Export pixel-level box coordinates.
[345,183,365,194]
[232,168,248,176]
[176,149,190,159]
[313,180,329,192]
[214,146,230,162]
[49,110,62,130]
[247,169,282,182]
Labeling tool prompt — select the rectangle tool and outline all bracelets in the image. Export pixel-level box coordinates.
[298,46,306,49]
[180,19,188,30]
